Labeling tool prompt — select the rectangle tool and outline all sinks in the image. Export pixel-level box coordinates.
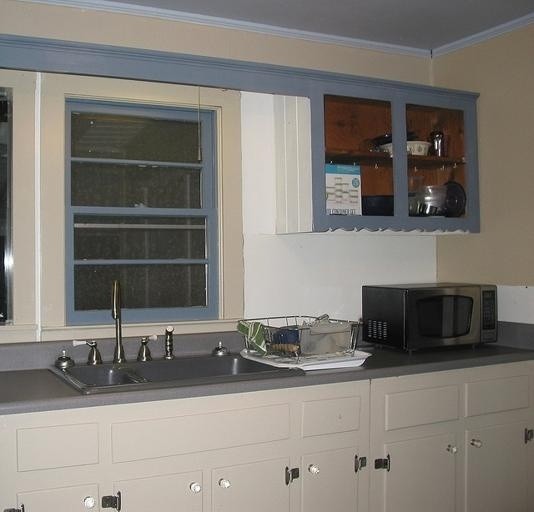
[126,351,305,396]
[47,360,139,405]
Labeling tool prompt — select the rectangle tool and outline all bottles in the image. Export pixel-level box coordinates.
[429,132,443,155]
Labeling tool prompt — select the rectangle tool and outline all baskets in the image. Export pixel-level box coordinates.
[238,316,359,360]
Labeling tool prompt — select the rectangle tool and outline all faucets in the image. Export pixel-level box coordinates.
[110,279,128,363]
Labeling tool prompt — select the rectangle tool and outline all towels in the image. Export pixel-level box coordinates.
[237,319,268,358]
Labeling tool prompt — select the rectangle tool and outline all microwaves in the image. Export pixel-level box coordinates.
[361,283,498,349]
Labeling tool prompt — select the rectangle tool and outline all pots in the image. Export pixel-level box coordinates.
[373,132,419,146]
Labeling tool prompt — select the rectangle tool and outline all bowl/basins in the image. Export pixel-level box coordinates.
[361,196,393,216]
[421,185,447,212]
[379,141,431,157]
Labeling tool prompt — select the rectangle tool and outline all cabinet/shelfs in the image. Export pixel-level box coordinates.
[298,372,373,511]
[102,378,298,512]
[374,349,533,511]
[0,393,102,511]
[270,69,482,240]
